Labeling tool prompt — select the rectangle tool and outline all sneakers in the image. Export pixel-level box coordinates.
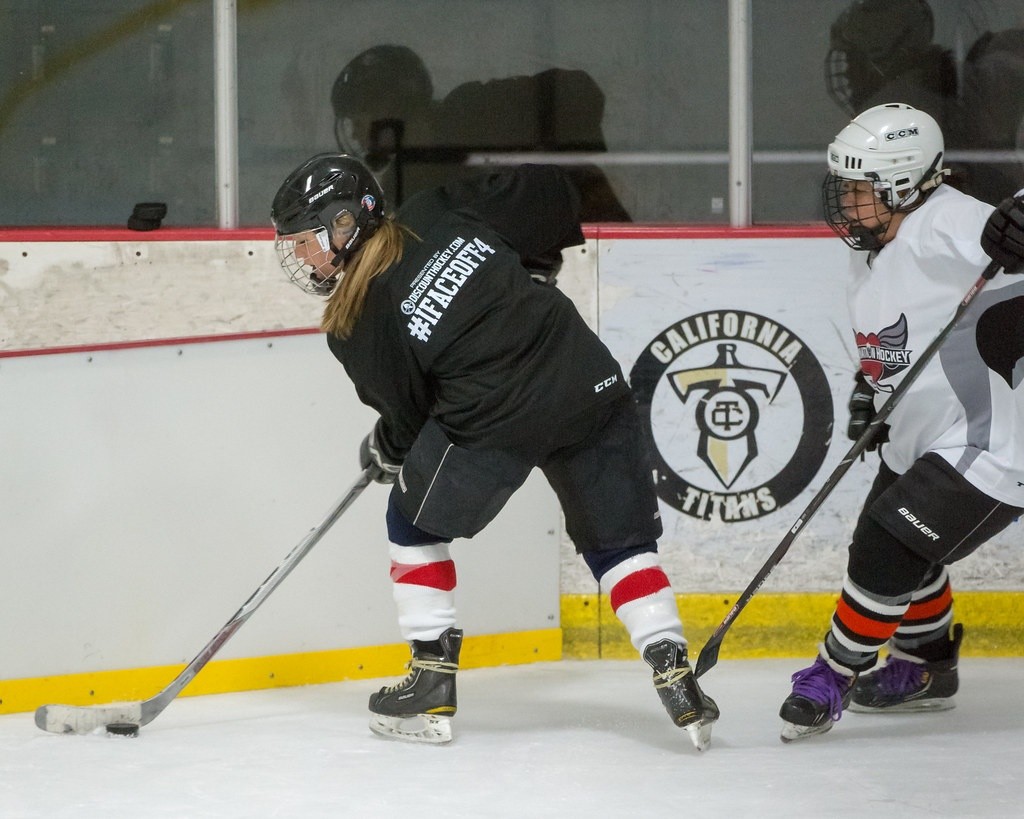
[369,627,460,748]
[779,649,856,746]
[848,628,969,716]
[640,635,717,750]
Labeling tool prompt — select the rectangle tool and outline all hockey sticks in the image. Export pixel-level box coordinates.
[34,463,383,736]
[690,260,1003,679]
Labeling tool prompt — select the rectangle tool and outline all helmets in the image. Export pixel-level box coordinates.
[327,59,436,172]
[822,102,951,254]
[273,156,389,302]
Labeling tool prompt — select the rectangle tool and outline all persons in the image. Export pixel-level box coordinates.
[779,101,1023,739]
[273,152,718,751]
[825,2,1023,206]
[332,44,633,224]
